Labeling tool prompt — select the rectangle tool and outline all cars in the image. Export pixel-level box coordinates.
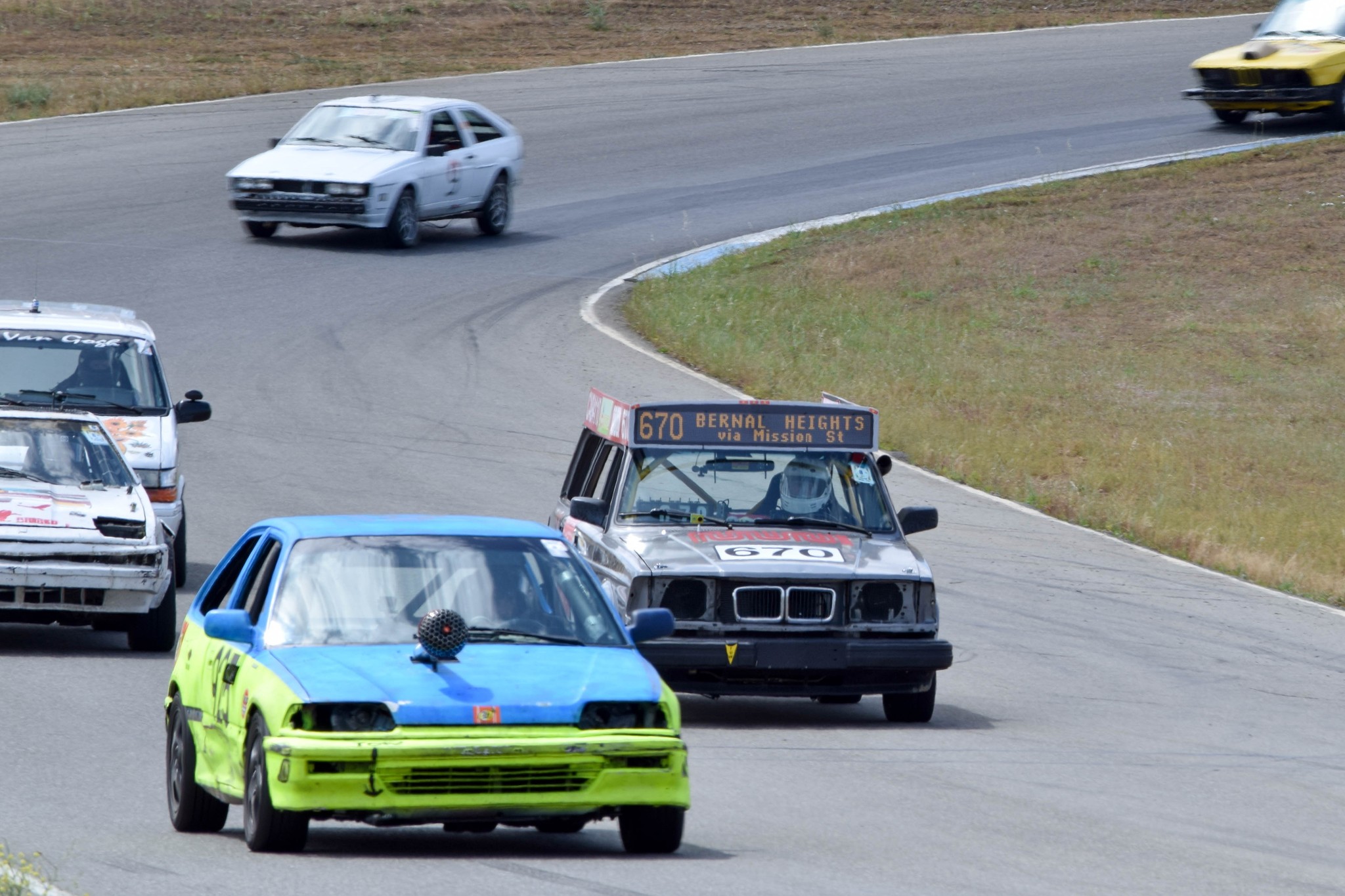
[163,513,692,858]
[0,300,212,586]
[0,409,179,652]
[549,386,952,723]
[1180,0,1345,133]
[226,94,522,250]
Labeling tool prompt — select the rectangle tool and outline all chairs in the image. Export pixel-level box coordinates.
[753,472,857,527]
[455,572,564,634]
[115,361,137,406]
[23,441,38,471]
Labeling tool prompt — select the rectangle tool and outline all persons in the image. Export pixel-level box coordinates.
[78,350,120,386]
[779,457,833,519]
[33,428,83,475]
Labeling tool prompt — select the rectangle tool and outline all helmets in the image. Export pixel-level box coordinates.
[76,343,121,388]
[779,456,832,515]
[21,435,75,478]
